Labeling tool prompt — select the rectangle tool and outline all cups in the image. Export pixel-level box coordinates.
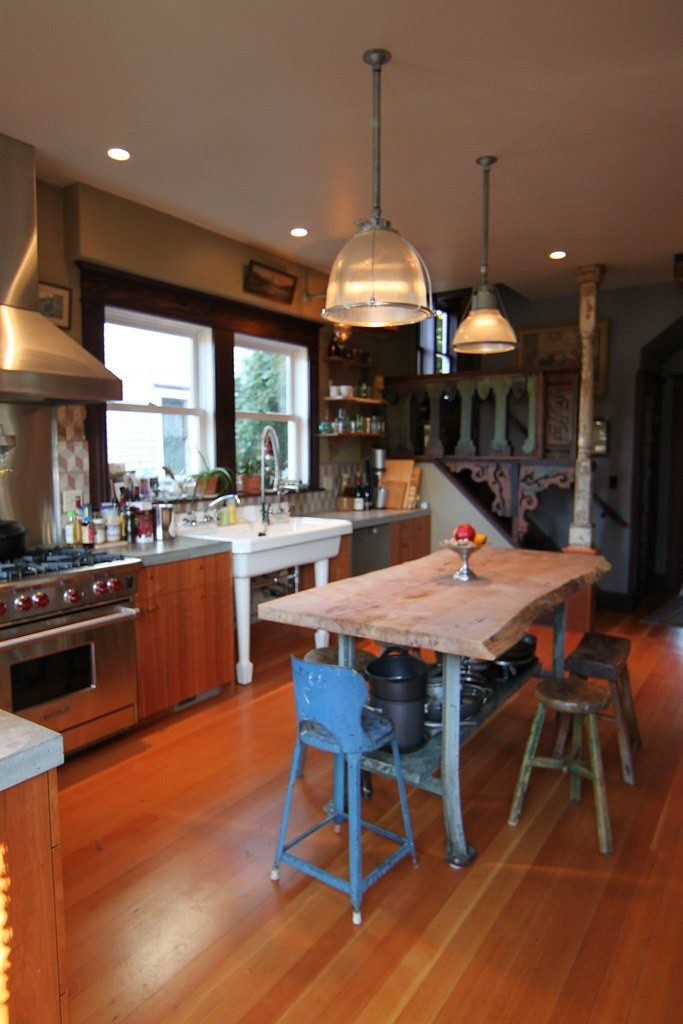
[328,384,355,398]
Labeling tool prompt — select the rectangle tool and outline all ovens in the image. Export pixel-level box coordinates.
[0,595,141,758]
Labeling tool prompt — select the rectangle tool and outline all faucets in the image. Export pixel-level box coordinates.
[257,425,299,535]
[202,494,241,524]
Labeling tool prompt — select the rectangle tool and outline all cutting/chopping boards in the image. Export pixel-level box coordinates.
[381,458,424,509]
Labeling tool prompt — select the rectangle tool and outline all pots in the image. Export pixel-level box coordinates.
[364,630,541,752]
[0,518,29,564]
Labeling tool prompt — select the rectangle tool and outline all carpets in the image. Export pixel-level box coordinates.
[640,590,683,628]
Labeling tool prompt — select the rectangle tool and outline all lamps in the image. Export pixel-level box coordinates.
[320,48,435,329]
[451,154,518,354]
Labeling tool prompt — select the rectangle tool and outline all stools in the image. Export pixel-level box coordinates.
[266,652,418,924]
[506,677,613,856]
[552,632,643,784]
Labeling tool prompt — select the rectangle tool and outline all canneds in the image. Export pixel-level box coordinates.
[92,502,120,543]
[139,476,160,497]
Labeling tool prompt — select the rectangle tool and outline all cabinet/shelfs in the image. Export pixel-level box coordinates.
[351,515,430,576]
[315,320,387,439]
[134,553,235,729]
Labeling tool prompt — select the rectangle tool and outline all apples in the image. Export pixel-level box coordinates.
[454,524,477,541]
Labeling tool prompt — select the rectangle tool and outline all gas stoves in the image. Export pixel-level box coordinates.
[0,544,144,630]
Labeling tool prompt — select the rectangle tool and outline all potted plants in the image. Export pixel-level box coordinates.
[186,445,236,508]
[237,456,286,495]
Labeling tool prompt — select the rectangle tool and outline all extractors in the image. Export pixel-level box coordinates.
[0,133,126,407]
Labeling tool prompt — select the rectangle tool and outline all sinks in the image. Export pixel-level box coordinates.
[189,519,353,553]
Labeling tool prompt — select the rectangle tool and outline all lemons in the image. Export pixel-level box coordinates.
[474,533,487,545]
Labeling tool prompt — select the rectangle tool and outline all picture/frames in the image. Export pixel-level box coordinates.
[592,416,611,458]
[243,259,299,304]
[38,280,73,332]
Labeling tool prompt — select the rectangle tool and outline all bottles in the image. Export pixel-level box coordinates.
[318,411,388,435]
[65,470,176,549]
[334,471,386,512]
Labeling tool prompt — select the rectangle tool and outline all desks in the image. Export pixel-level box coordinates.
[258,545,612,870]
